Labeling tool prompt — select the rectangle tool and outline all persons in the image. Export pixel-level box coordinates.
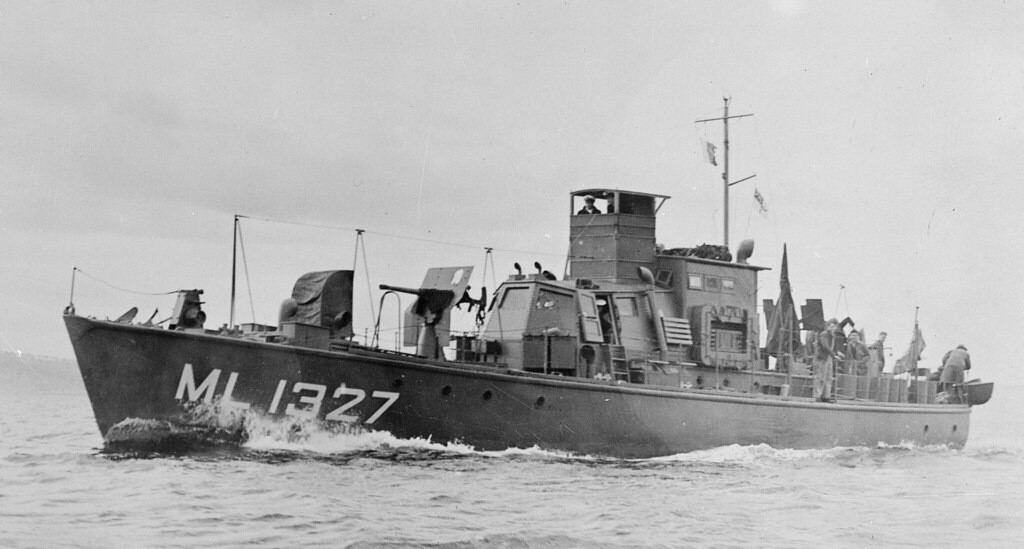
[940,343,972,406]
[842,329,869,376]
[812,318,842,404]
[868,331,889,374]
[603,191,615,214]
[576,193,601,214]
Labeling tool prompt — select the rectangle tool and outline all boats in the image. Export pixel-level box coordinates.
[61,92,994,463]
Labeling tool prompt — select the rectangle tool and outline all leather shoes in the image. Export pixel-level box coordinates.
[816,397,836,403]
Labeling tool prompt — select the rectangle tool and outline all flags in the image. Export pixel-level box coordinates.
[706,141,719,167]
[892,328,926,376]
[754,187,768,213]
